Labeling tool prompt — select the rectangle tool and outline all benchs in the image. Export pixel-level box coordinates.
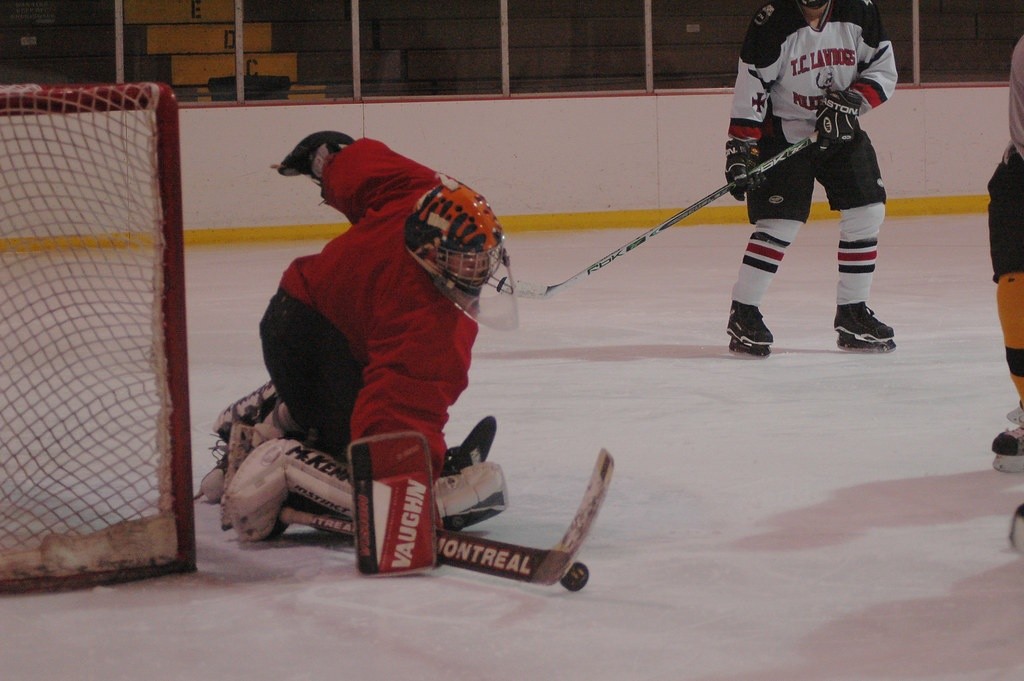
[0,0,766,102]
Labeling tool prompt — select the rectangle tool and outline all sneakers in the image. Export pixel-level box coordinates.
[726,299,773,357]
[834,302,896,353]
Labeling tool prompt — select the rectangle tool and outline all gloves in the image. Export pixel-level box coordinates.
[279,131,356,199]
[724,138,766,202]
[813,90,863,161]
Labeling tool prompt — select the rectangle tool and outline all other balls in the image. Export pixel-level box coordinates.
[559,561,589,591]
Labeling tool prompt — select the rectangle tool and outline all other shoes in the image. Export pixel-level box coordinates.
[1010,504,1024,552]
[991,404,1024,474]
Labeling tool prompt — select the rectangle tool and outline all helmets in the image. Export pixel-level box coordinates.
[404,173,502,296]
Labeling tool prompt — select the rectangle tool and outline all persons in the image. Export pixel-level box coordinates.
[195,130,517,578]
[988,35,1024,456]
[725,0,898,348]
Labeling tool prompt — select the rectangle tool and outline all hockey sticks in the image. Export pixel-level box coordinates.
[278,446,616,587]
[497,131,821,300]
